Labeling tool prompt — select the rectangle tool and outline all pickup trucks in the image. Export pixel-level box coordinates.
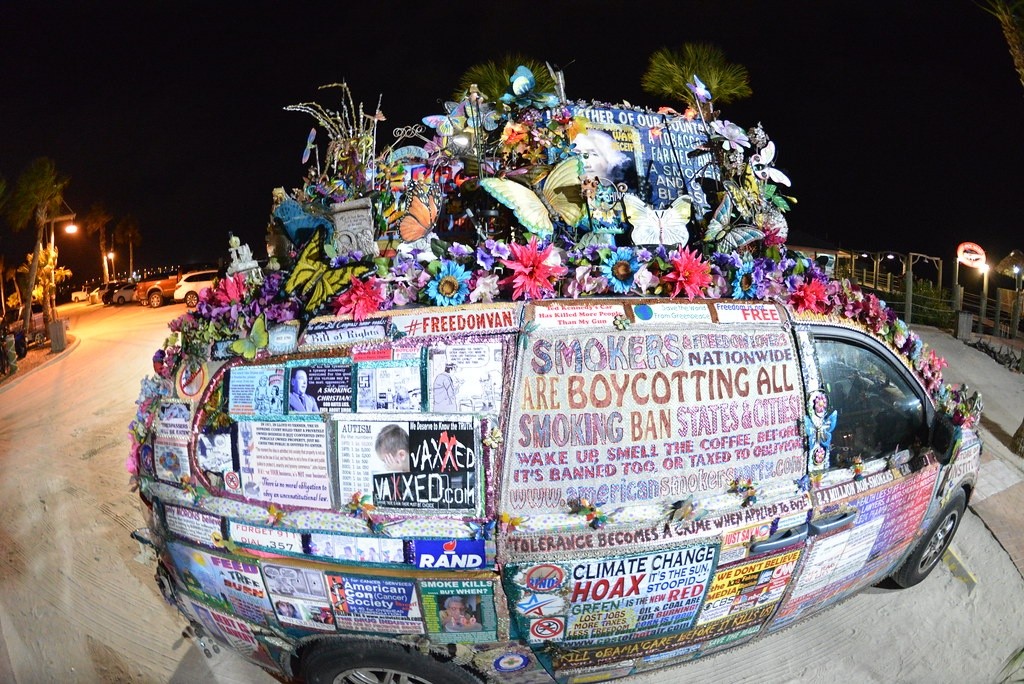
[71,286,88,302]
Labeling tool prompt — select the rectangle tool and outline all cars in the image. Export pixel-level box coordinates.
[174,267,218,307]
[133,272,184,307]
[88,282,134,305]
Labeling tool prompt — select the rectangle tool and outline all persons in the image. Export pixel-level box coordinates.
[440,595,467,630]
[374,424,410,472]
[289,370,320,413]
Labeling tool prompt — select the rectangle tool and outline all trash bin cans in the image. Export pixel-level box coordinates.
[48,319,66,353]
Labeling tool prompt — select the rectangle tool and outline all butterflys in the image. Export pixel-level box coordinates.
[315,147,366,204]
[420,101,466,137]
[464,103,501,130]
[281,225,376,343]
[480,154,587,241]
[398,179,440,243]
[227,312,271,362]
[202,387,236,436]
[482,427,504,449]
[750,140,791,188]
[622,193,692,250]
[802,409,838,450]
[722,159,767,222]
[498,65,560,111]
[702,192,766,254]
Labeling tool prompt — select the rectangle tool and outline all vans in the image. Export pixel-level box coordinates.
[125,59,984,684]
